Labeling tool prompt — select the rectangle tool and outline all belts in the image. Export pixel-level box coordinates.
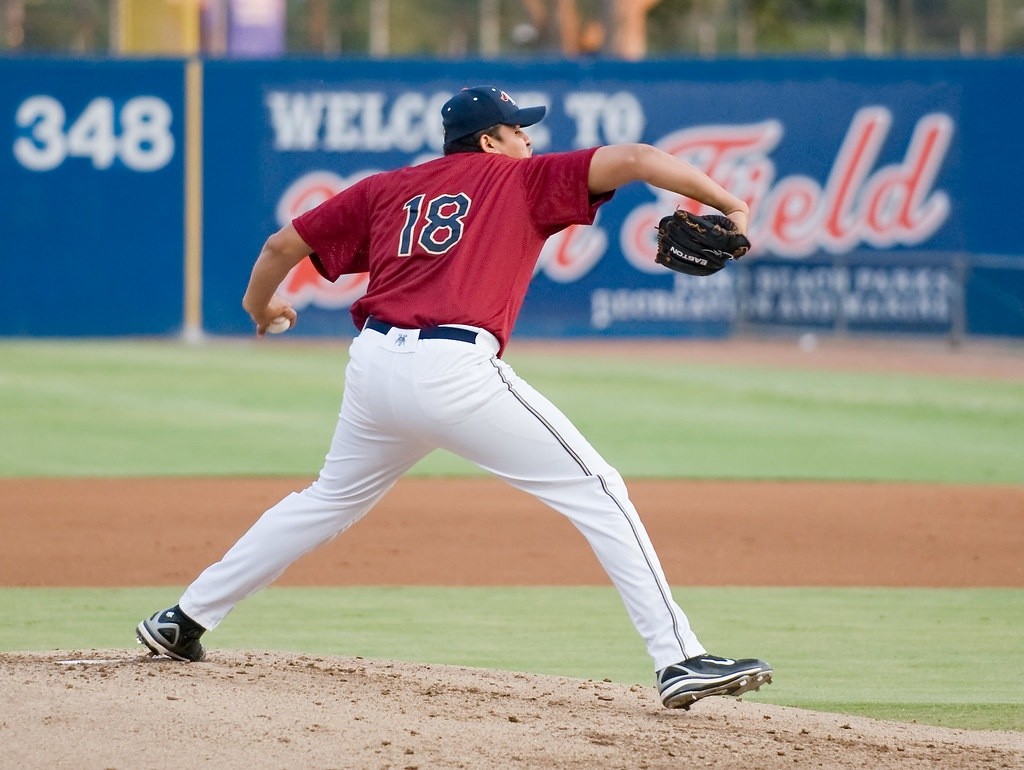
[364,315,479,344]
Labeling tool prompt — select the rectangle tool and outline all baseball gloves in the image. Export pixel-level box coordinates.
[652,213,750,281]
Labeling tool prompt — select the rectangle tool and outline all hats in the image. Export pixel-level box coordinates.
[439,86,549,142]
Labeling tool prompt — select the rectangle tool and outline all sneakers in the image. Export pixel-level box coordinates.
[135,604,208,663]
[657,655,777,707]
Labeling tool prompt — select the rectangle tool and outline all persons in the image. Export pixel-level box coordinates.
[135,85,772,708]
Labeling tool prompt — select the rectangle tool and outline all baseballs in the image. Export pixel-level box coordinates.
[266,316,290,335]
